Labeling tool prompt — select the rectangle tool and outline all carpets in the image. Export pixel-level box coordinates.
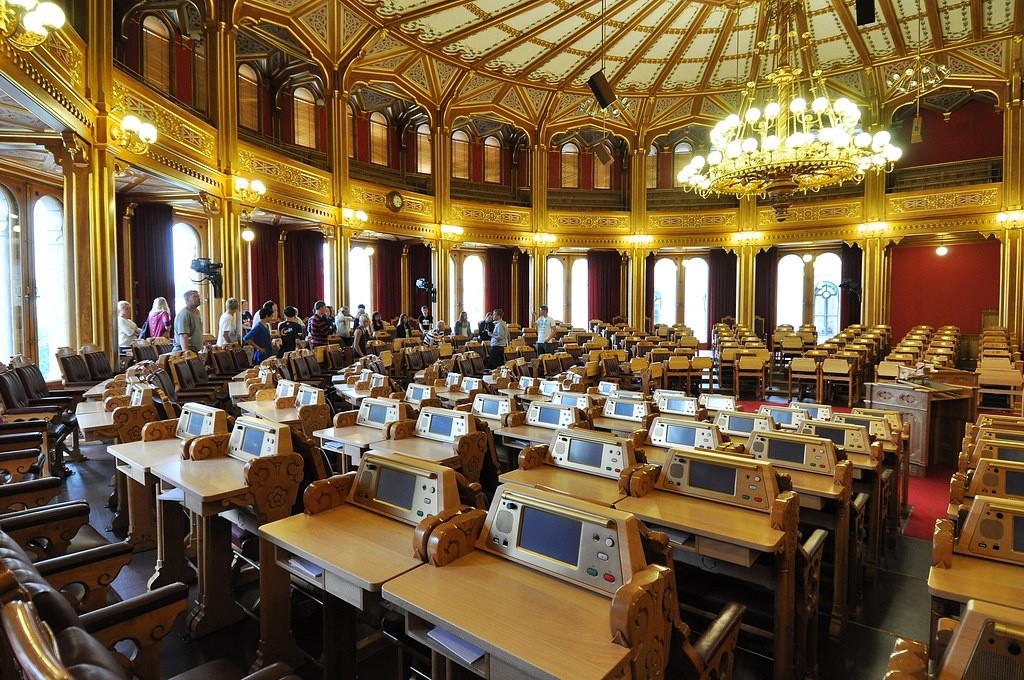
[735,398,1024,546]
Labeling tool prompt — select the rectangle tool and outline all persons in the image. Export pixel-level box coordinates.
[488,308,508,370]
[534,306,557,356]
[396,314,412,366]
[149,297,171,339]
[455,312,471,338]
[422,321,445,348]
[418,306,433,333]
[171,290,203,355]
[307,300,335,349]
[478,312,495,342]
[117,300,142,366]
[334,304,384,363]
[216,298,238,346]
[242,299,278,364]
[278,306,306,359]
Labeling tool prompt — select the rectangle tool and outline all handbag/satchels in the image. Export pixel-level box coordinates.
[137,313,149,340]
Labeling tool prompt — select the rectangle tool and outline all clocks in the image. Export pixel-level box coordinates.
[386,191,405,213]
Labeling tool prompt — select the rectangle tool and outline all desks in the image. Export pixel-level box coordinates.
[75,355,907,679]
[883,421,1024,679]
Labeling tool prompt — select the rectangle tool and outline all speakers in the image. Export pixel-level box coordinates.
[911,117,925,143]
[587,70,617,110]
[856,0,876,26]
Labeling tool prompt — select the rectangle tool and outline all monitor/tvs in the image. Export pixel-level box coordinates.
[185,370,884,570]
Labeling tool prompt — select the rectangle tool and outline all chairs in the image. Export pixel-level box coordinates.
[0,365,304,680]
[0,308,1024,410]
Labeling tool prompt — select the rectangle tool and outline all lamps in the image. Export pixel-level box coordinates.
[441,225,465,244]
[730,230,764,248]
[532,233,557,251]
[622,235,654,252]
[112,115,158,155]
[0,0,66,52]
[236,178,266,241]
[343,208,375,256]
[996,211,1024,231]
[672,0,950,256]
